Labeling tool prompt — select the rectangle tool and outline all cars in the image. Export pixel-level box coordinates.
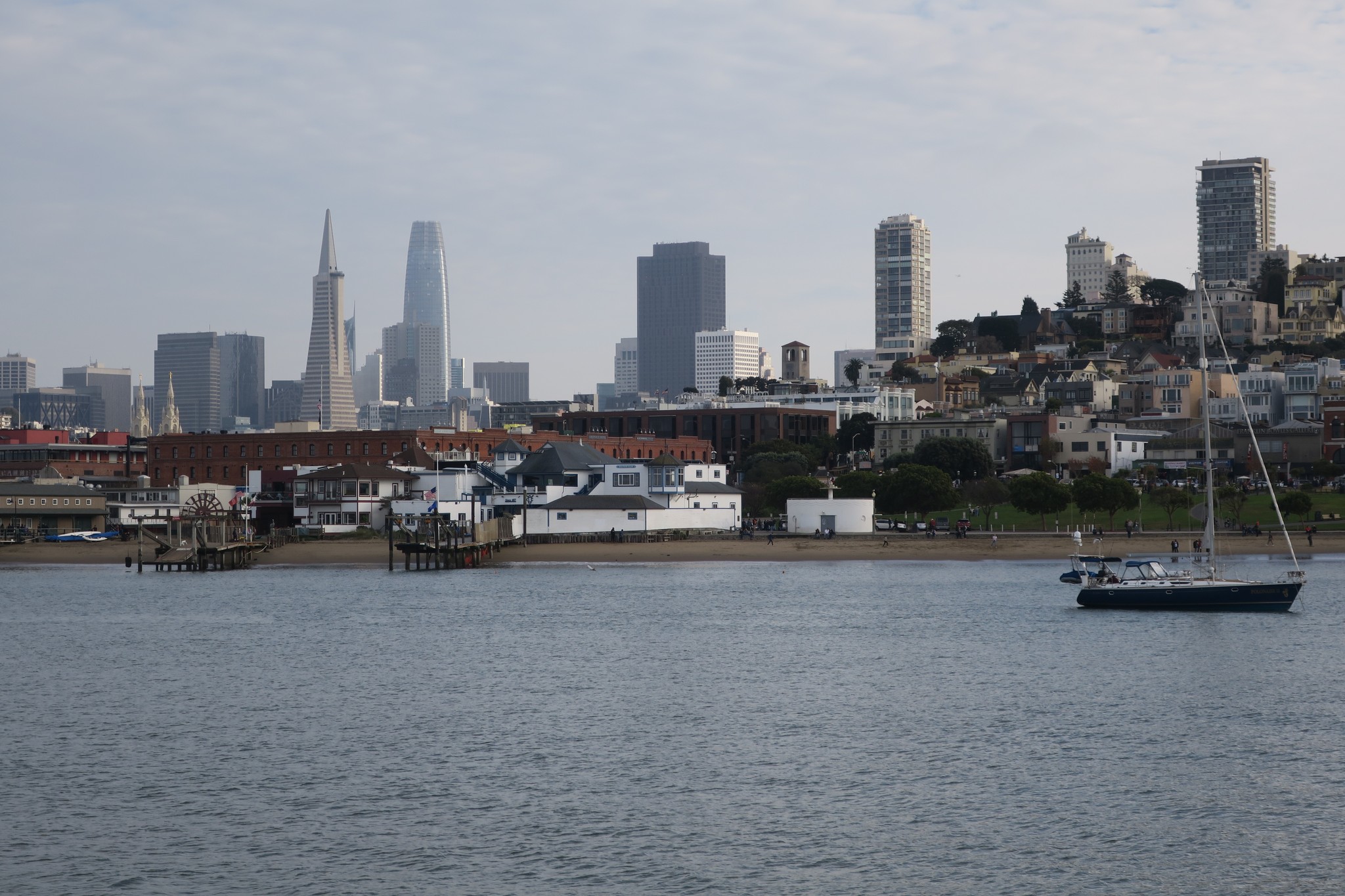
[916,520,926,530]
[1059,479,1075,486]
[1327,481,1340,487]
[1127,479,1169,487]
[1249,478,1289,488]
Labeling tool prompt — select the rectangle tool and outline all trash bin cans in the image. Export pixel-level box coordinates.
[1193,489,1198,495]
[1315,511,1321,521]
[1232,519,1236,530]
[240,537,244,542]
[1255,483,1258,489]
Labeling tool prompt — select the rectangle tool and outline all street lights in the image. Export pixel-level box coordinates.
[1311,466,1314,486]
[10,493,18,545]
[826,472,830,485]
[968,504,971,523]
[871,492,876,538]
[1138,488,1144,533]
[957,471,960,487]
[974,472,977,484]
[1278,465,1280,490]
[1256,472,1259,496]
[1047,460,1058,482]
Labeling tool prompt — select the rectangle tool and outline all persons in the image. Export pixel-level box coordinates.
[256,491,293,500]
[618,529,623,543]
[611,527,615,542]
[875,505,980,538]
[1093,477,1336,554]
[233,529,237,542]
[990,534,997,548]
[739,517,833,540]
[883,536,888,547]
[0,522,123,536]
[767,532,776,546]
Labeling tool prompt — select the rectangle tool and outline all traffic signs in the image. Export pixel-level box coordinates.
[1164,461,1186,469]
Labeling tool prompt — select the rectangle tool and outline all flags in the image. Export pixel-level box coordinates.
[655,389,658,394]
[229,491,256,507]
[428,500,437,512]
[317,398,321,412]
[424,486,436,500]
[662,389,668,394]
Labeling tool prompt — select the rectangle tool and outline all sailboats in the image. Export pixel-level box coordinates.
[1077,271,1309,611]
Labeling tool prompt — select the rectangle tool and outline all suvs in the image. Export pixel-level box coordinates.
[955,519,971,531]
[1171,479,1187,487]
[935,517,950,531]
[875,519,906,531]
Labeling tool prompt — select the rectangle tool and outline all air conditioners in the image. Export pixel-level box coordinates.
[293,464,300,470]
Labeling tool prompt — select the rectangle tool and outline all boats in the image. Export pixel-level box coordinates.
[82,535,108,542]
